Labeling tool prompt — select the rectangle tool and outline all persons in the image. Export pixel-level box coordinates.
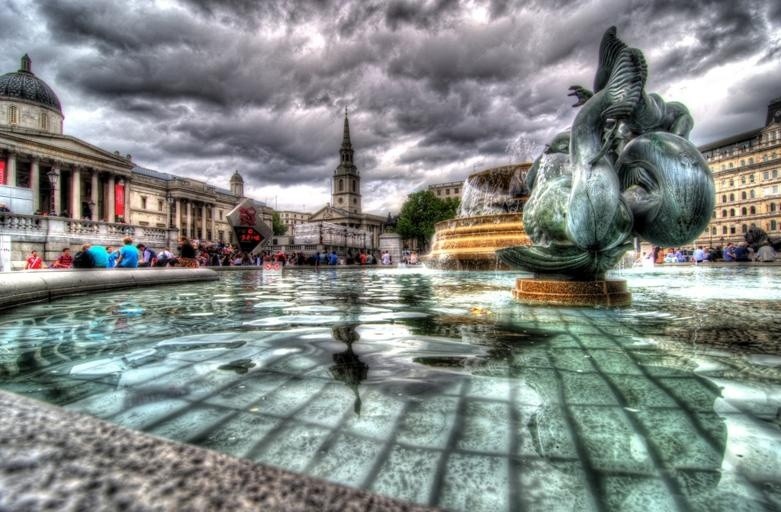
[0,201,418,270]
[642,223,776,264]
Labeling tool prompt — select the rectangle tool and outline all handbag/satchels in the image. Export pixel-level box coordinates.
[73,252,92,268]
